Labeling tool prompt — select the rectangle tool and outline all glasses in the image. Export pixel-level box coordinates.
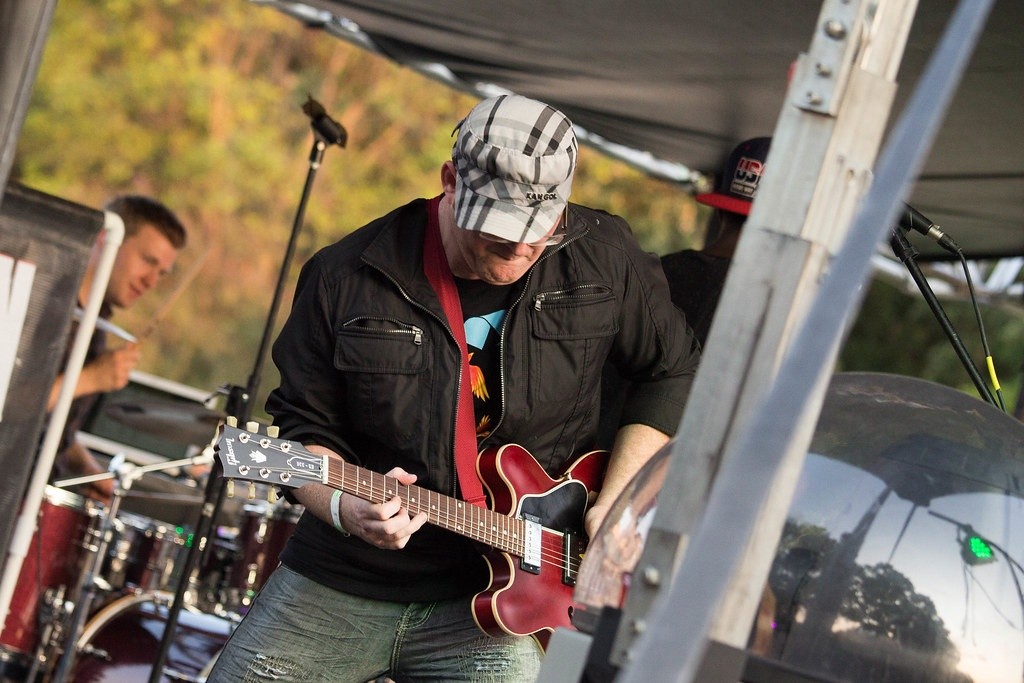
[476,230,569,249]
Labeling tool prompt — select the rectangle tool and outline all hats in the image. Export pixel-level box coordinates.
[691,135,773,217]
[448,92,581,244]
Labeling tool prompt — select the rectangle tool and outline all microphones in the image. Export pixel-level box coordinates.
[899,203,962,255]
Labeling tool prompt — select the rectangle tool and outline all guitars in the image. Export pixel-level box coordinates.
[213,412,613,658]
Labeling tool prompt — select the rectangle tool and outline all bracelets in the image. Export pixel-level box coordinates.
[331,489,351,538]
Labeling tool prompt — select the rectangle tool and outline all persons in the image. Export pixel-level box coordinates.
[662,135,772,350]
[21,193,189,502]
[208,94,702,683]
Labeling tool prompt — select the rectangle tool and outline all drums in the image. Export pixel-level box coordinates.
[0,483,290,683]
